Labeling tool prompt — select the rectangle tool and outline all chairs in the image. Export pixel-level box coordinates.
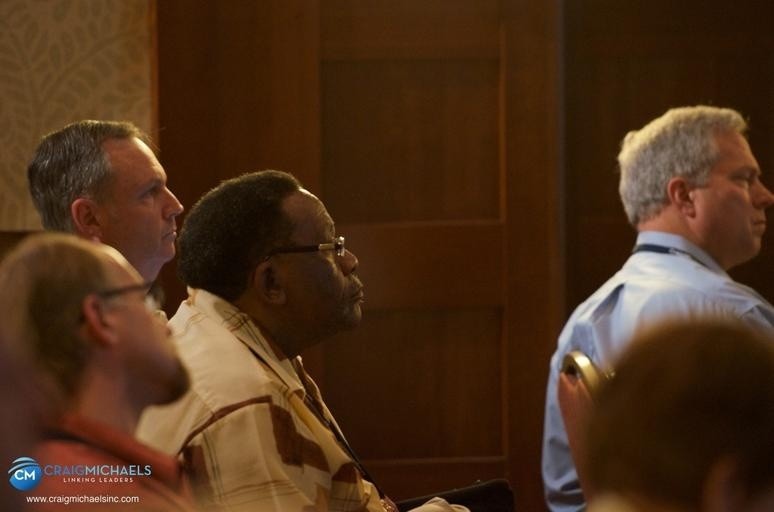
[556,350,603,503]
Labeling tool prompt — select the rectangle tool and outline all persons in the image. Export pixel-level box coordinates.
[25,119,184,301]
[581,311,774,512]
[541,103,774,512]
[132,170,472,511]
[0,232,206,511]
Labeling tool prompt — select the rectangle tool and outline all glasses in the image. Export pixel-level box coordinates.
[77,279,168,327]
[262,235,346,260]
